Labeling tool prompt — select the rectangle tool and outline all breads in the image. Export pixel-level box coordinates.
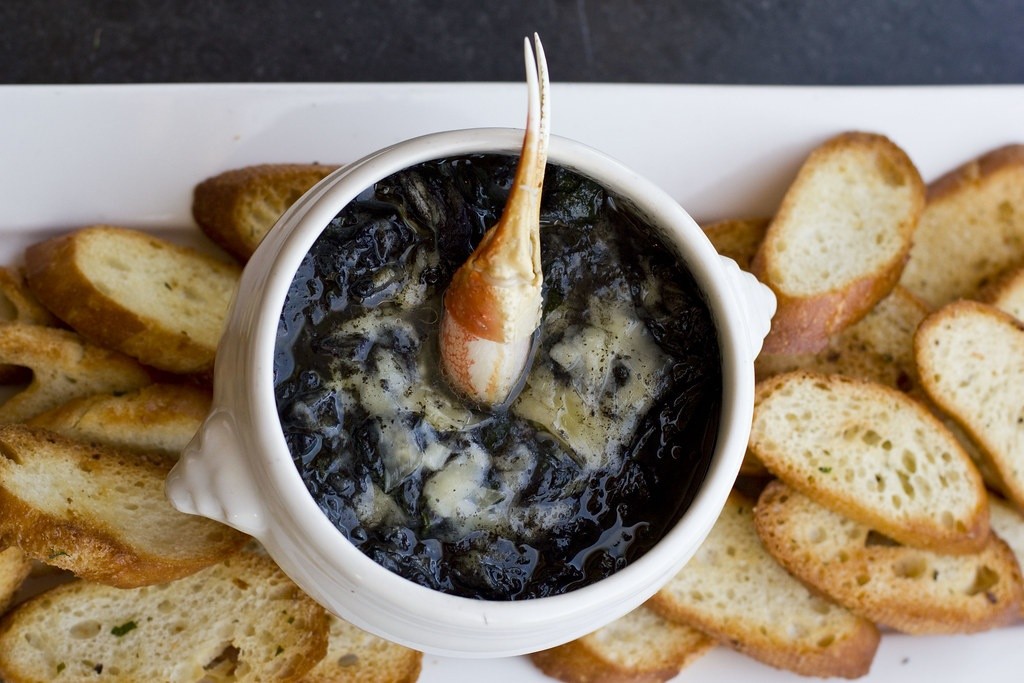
[0,120,421,683]
[527,129,1024,683]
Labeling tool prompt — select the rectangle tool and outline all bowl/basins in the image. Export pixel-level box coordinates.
[164,128,777,659]
